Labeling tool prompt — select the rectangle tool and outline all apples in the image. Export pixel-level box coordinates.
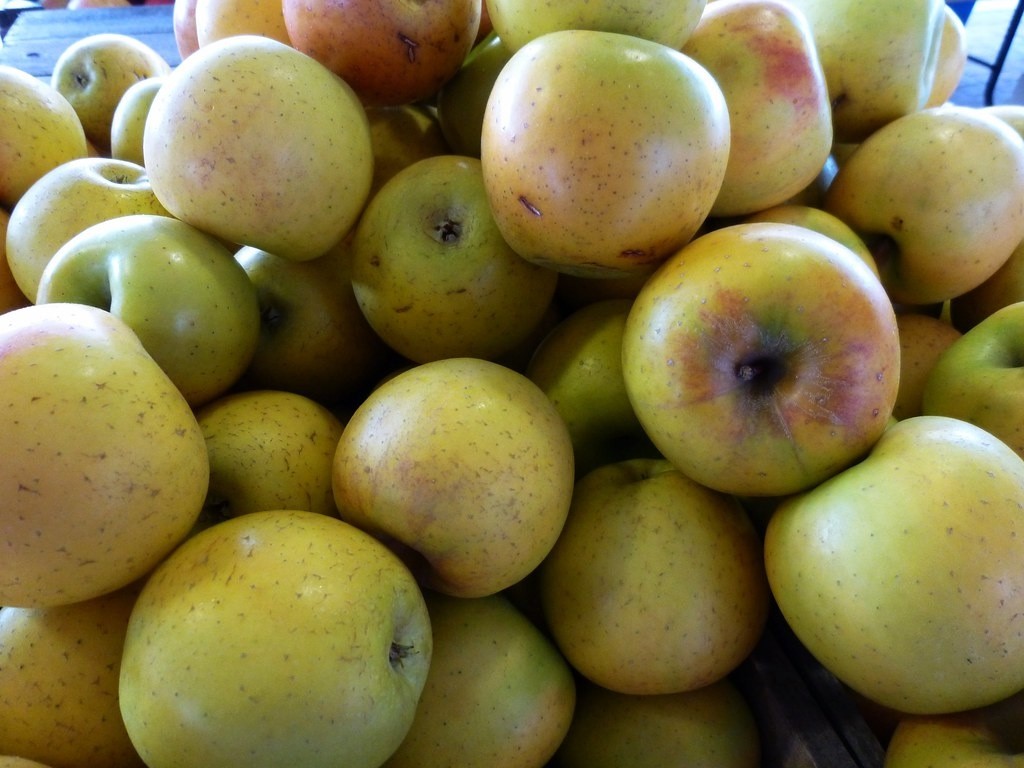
[0,0,1024,768]
